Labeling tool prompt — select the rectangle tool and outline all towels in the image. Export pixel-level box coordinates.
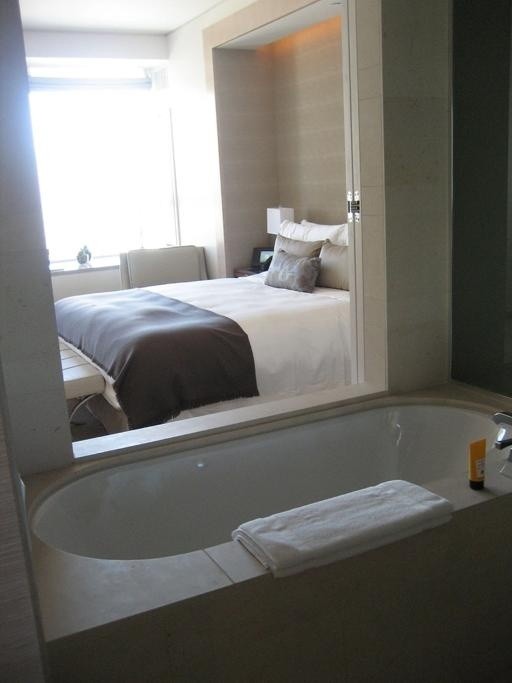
[231,479,453,578]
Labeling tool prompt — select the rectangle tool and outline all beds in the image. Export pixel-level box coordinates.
[53,271,354,434]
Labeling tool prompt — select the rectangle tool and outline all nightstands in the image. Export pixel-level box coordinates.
[234,266,256,277]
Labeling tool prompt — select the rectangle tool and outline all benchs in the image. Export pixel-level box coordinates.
[58,340,107,424]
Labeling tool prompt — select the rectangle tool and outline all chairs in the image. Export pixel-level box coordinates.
[119,245,210,291]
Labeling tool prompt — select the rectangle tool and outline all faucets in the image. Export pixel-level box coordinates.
[489,408,512,478]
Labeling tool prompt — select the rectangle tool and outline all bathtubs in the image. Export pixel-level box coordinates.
[22,391,512,648]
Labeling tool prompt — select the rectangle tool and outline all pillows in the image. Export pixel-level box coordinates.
[264,219,353,293]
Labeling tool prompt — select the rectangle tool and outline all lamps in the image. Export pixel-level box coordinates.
[265,206,296,237]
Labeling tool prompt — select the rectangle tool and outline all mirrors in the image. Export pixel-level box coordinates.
[13,0,365,461]
